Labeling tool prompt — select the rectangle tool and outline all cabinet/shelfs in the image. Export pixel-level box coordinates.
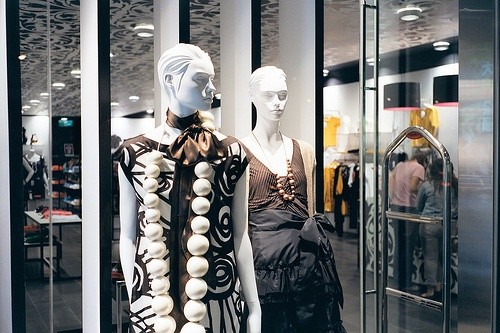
[52,156,82,206]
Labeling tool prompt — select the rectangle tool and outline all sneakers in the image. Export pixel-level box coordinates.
[419,293,434,300]
[434,288,442,297]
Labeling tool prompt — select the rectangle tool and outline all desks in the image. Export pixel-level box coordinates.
[24,209,82,280]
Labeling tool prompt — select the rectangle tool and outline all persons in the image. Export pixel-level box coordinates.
[416,157,457,298]
[390,153,428,291]
[24,149,48,198]
[117,43,262,333]
[238,66,346,333]
[23,155,34,201]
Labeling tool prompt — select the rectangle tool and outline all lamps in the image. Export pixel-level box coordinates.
[433,41,450,51]
[397,5,423,21]
[432,75,459,106]
[365,57,381,67]
[383,73,421,111]
[22,19,154,113]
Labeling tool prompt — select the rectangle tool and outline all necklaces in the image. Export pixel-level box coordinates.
[251,130,295,201]
